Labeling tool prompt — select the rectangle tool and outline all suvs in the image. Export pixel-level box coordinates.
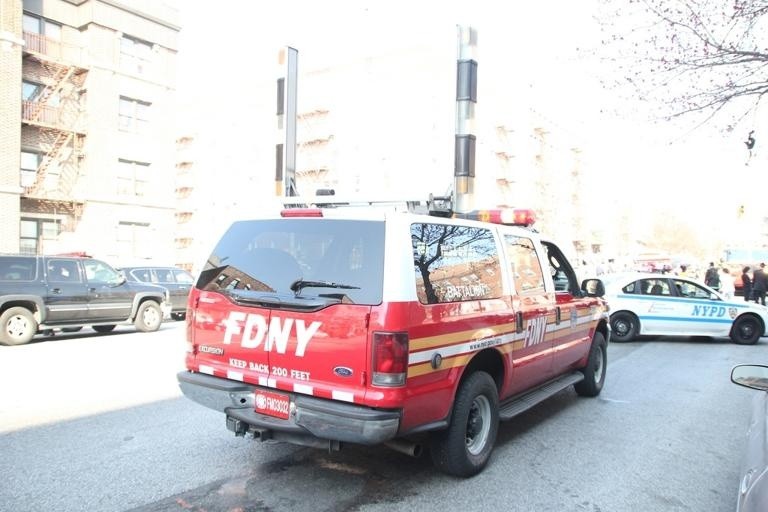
[0,254,171,347]
[176,204,611,478]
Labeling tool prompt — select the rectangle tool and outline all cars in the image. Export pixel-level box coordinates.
[620,247,677,274]
[598,273,768,345]
[710,261,753,296]
[95,265,197,324]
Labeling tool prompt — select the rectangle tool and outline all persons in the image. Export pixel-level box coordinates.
[752,262,768,305]
[591,257,736,307]
[741,266,752,302]
